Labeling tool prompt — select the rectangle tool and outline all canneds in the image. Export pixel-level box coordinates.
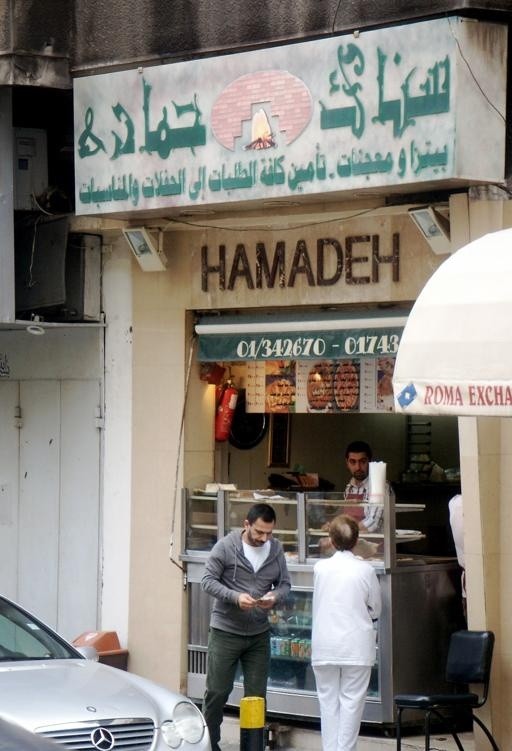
[270,635,312,659]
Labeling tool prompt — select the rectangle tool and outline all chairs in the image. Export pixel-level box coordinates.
[391,630,500,750]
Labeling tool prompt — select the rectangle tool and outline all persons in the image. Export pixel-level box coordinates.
[196,500,294,751]
[306,517,382,751]
[321,441,396,557]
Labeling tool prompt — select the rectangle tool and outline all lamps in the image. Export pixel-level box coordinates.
[408,203,454,256]
[121,226,168,273]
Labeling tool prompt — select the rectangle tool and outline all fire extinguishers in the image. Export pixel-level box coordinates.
[215,379,238,443]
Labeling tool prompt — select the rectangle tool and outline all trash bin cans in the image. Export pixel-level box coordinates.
[71,630,128,671]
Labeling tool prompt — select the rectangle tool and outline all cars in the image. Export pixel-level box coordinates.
[1,592,218,751]
